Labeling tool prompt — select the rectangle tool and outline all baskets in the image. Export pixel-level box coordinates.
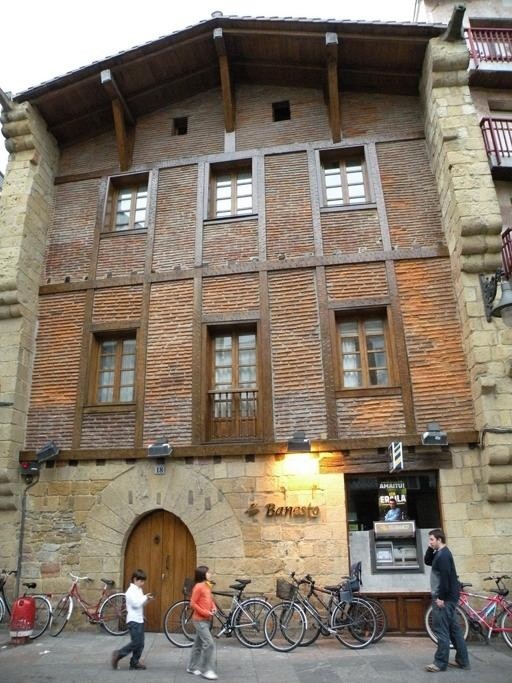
[182,576,194,595]
[276,578,293,600]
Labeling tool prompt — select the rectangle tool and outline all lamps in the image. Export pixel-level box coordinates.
[147,433,175,458]
[286,429,311,453]
[37,436,68,463]
[420,422,448,445]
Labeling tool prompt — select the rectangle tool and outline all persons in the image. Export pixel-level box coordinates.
[424,529,469,672]
[384,498,400,521]
[112,568,155,669]
[186,566,218,680]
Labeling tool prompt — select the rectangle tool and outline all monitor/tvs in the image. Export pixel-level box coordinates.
[376,547,392,563]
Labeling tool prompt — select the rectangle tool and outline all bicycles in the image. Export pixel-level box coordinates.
[49,571,131,637]
[1,566,53,639]
[163,560,386,650]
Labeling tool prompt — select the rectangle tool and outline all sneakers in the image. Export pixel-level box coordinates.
[129,664,145,670]
[202,671,219,680]
[425,663,441,672]
[188,666,200,676]
[112,650,121,670]
[449,661,471,669]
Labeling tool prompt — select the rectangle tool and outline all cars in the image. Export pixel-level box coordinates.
[423,574,512,648]
